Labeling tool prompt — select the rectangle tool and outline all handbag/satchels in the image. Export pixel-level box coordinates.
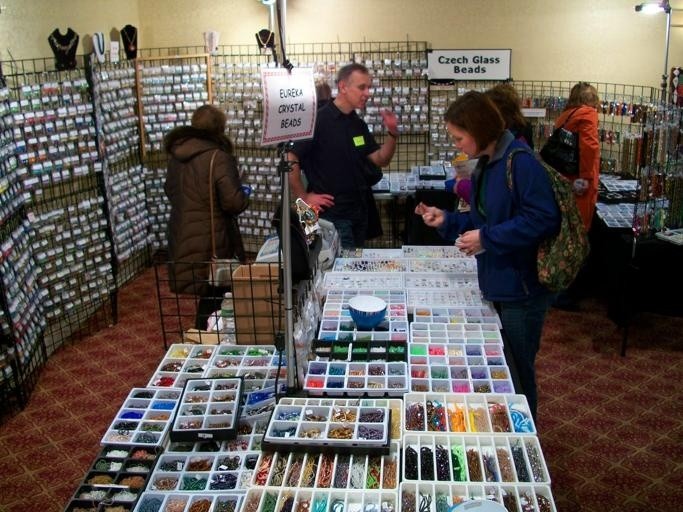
[271,203,323,281]
[539,107,583,176]
[208,258,242,288]
[353,157,383,186]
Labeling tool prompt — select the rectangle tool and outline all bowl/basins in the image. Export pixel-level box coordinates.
[451,499,508,512]
[349,294,387,329]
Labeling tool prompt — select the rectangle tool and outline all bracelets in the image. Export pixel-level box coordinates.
[387,131,397,138]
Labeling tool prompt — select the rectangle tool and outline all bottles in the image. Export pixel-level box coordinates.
[221,293,236,346]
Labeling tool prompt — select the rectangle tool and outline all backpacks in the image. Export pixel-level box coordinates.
[505,147,591,293]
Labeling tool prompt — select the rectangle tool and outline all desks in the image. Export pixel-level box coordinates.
[404,165,683,359]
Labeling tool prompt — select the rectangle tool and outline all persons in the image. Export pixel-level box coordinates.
[287,63,398,251]
[486,85,534,151]
[415,89,560,422]
[314,81,332,111]
[163,104,250,330]
[539,81,600,312]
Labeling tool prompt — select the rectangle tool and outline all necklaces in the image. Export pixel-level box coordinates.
[50,28,137,59]
[257,31,272,48]
